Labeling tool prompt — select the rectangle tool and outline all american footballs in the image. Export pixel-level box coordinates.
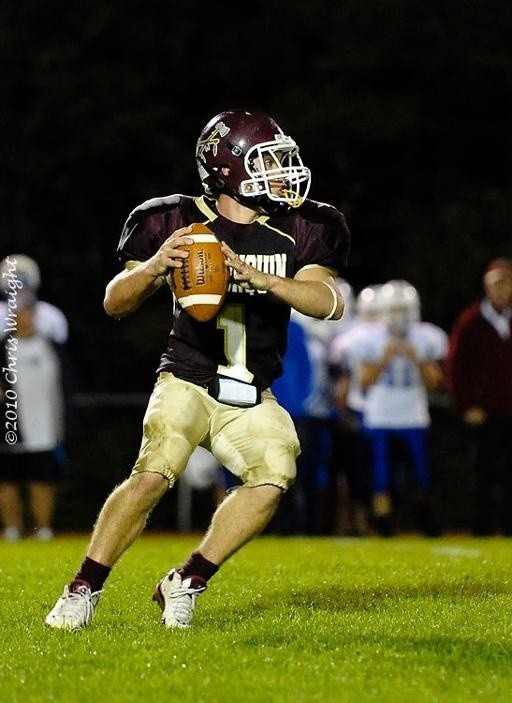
[172,222,230,322]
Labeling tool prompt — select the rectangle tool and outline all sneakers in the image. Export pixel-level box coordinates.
[149,565,211,630]
[40,578,106,634]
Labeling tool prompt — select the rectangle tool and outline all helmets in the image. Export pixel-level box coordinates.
[355,278,422,312]
[191,108,296,218]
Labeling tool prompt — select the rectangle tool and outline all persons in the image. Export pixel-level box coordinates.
[43,110,352,631]
[0,253,69,542]
[144,257,511,539]
